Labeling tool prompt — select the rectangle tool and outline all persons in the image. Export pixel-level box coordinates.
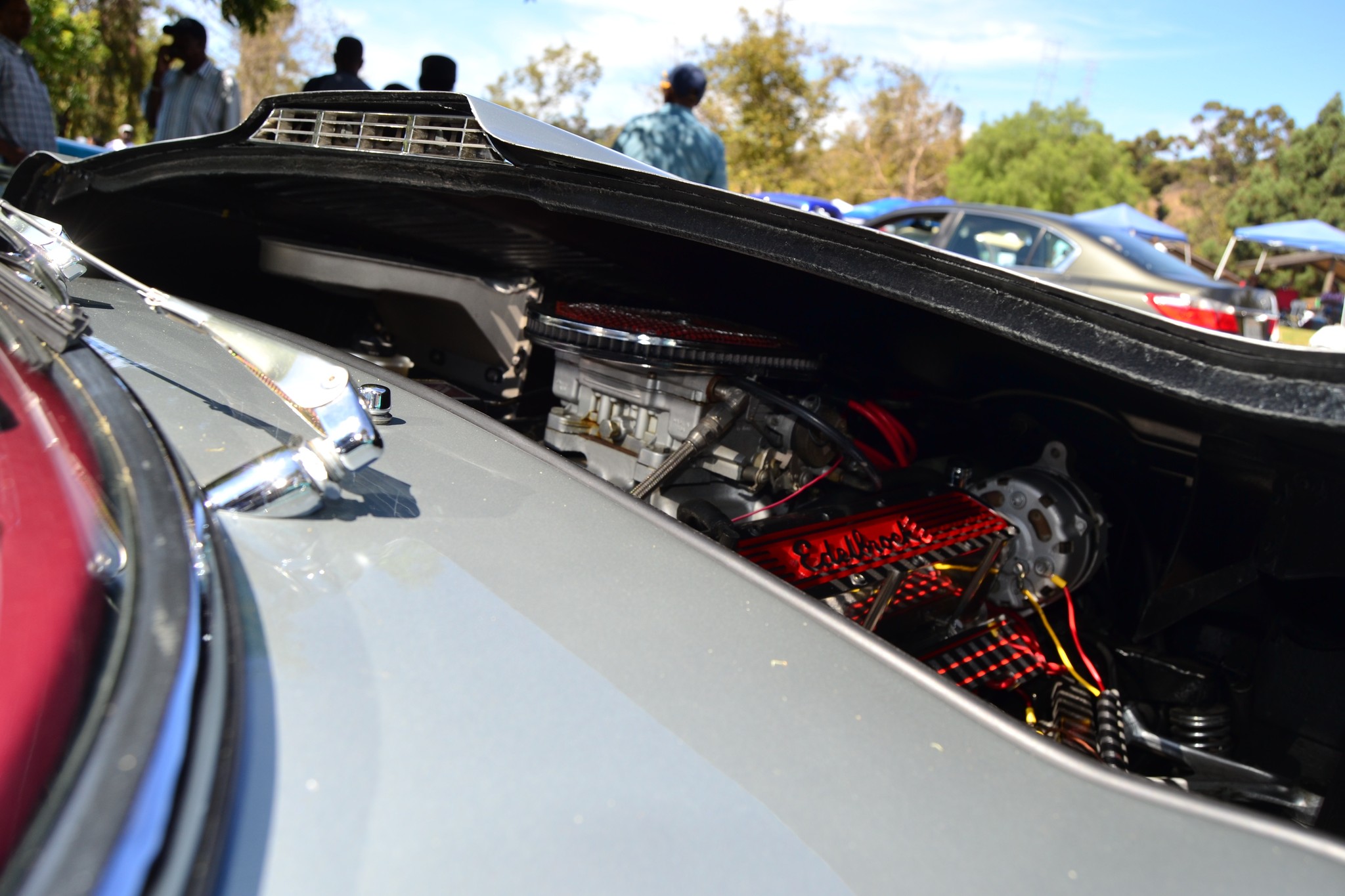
[609,66,726,193]
[105,124,138,152]
[139,18,240,141]
[0,0,57,169]
[420,55,457,91]
[291,37,378,140]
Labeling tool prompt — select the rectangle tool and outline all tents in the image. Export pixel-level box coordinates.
[1070,203,1192,264]
[1213,216,1345,296]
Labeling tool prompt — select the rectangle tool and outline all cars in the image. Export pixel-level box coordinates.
[0,76,1345,895]
[755,190,1281,347]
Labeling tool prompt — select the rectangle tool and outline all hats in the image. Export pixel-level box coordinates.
[163,18,206,47]
[666,65,707,102]
